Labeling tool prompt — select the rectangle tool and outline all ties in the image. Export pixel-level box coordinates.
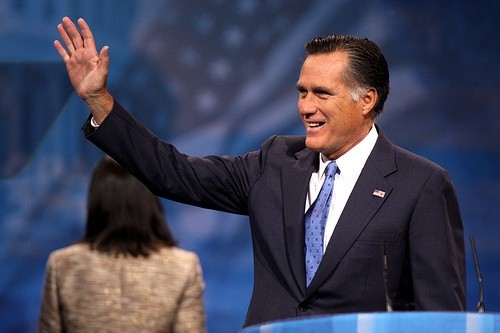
[304,160,340,286]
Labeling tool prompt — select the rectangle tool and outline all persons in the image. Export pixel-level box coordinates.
[54,16,467,329]
[40,153,207,333]
